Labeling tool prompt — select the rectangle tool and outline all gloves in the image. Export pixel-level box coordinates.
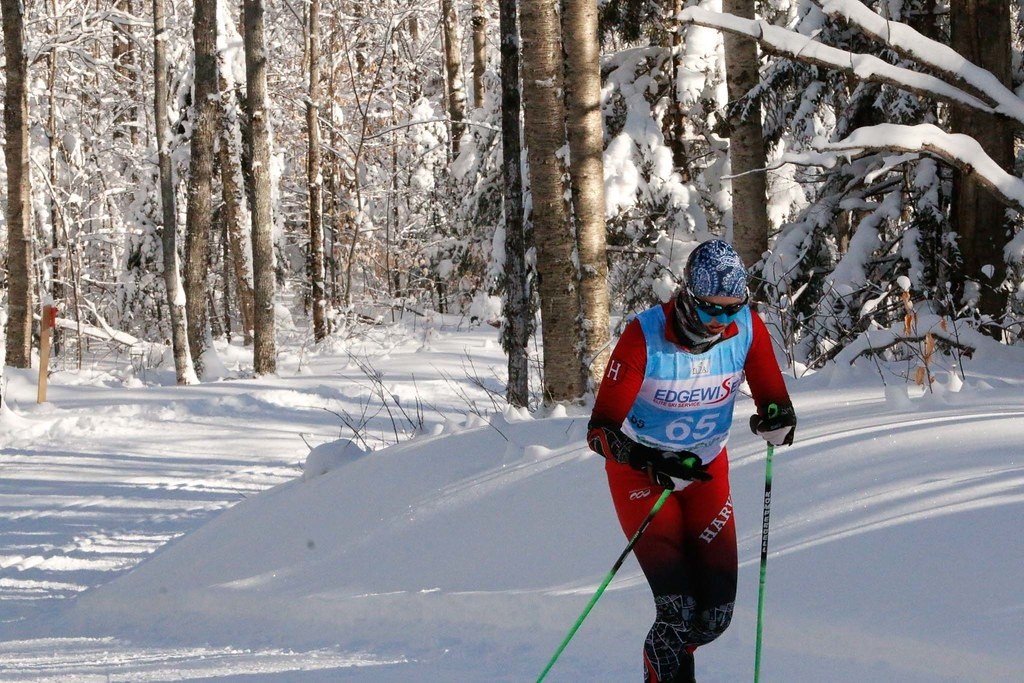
[630,443,713,492]
[750,407,796,446]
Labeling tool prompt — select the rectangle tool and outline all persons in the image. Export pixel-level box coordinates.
[587,239,797,682]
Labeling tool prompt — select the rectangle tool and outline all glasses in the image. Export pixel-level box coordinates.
[686,287,750,317]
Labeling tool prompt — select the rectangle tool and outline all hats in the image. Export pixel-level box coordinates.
[686,239,746,301]
